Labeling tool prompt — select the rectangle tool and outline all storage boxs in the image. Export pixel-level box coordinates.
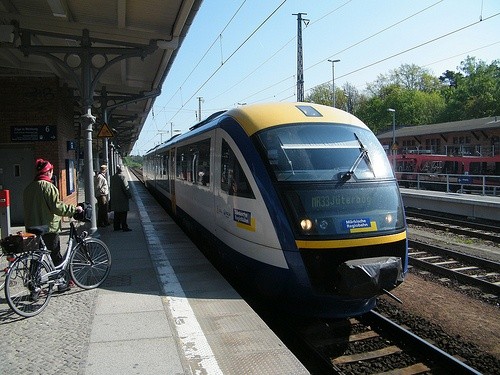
[0,235,40,255]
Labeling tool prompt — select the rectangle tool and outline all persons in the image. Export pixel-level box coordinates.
[109,164,134,232]
[23,159,83,302]
[94,165,112,228]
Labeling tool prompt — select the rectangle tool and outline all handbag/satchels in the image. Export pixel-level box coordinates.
[74,202,92,223]
[0,231,40,254]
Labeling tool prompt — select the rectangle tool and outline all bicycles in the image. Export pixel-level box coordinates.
[1,209,112,319]
[0,246,17,293]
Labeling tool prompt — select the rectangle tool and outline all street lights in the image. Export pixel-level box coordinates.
[327,59,341,108]
[387,107,396,170]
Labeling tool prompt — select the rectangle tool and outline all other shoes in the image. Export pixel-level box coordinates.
[123,227,132,232]
[31,289,48,299]
[113,227,121,230]
[57,283,70,292]
[96,223,110,228]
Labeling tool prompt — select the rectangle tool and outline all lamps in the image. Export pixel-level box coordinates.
[67,142,76,151]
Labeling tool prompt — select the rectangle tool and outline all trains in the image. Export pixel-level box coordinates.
[142,101,409,319]
[387,154,500,196]
